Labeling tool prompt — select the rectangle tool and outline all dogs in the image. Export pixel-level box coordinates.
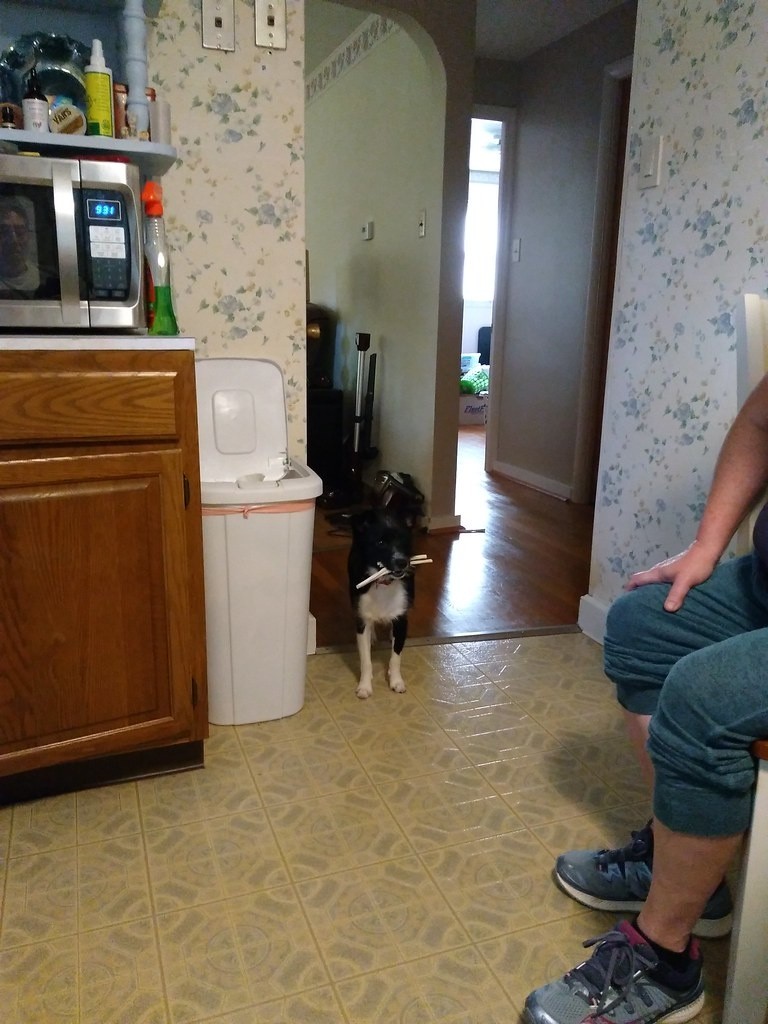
[345,505,416,699]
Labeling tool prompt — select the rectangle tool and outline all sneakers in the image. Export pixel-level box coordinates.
[524,923,705,1023]
[555,820,733,938]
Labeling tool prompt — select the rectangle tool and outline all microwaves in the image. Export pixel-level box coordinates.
[0,153,145,333]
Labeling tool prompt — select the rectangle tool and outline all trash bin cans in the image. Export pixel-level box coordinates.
[197,354,326,729]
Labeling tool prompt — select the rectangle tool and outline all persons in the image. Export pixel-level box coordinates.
[0,192,42,291]
[516,372,767,1024]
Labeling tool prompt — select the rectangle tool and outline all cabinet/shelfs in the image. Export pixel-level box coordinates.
[0,333,209,790]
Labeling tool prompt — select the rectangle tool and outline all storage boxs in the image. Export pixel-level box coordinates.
[458,393,486,425]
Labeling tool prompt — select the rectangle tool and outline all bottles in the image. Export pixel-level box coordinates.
[139,182,181,336]
[0,39,171,144]
